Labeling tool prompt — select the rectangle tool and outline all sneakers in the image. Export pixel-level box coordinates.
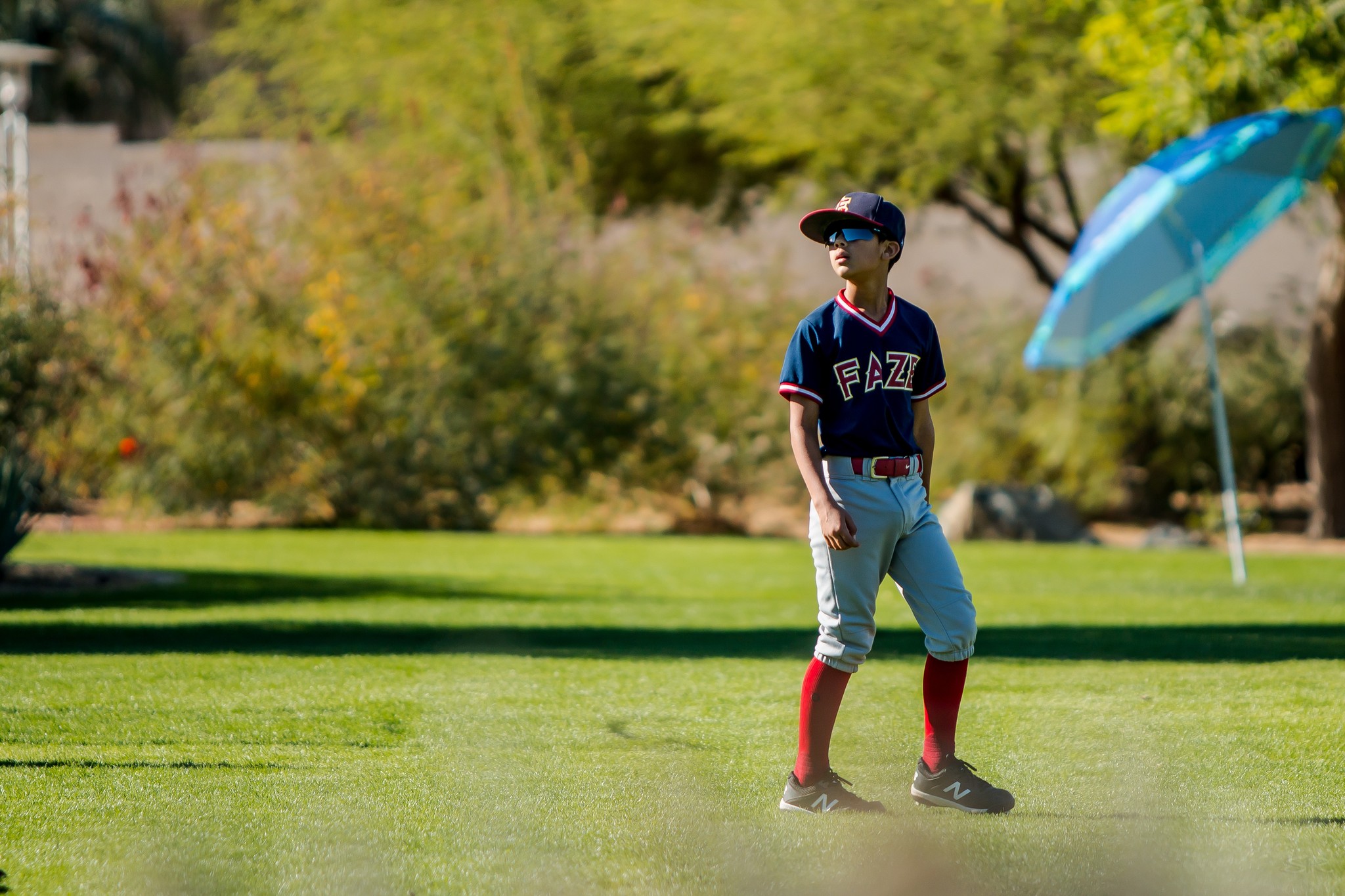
[911,753,1015,815]
[778,767,885,814]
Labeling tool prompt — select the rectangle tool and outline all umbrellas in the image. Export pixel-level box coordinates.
[1022,106,1345,585]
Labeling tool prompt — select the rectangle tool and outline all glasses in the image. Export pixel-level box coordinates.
[823,220,882,245]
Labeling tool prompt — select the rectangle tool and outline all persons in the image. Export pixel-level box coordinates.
[777,191,1015,816]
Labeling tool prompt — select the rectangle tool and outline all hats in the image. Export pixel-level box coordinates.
[799,191,906,254]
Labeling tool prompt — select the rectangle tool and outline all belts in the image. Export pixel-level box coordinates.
[851,454,923,479]
